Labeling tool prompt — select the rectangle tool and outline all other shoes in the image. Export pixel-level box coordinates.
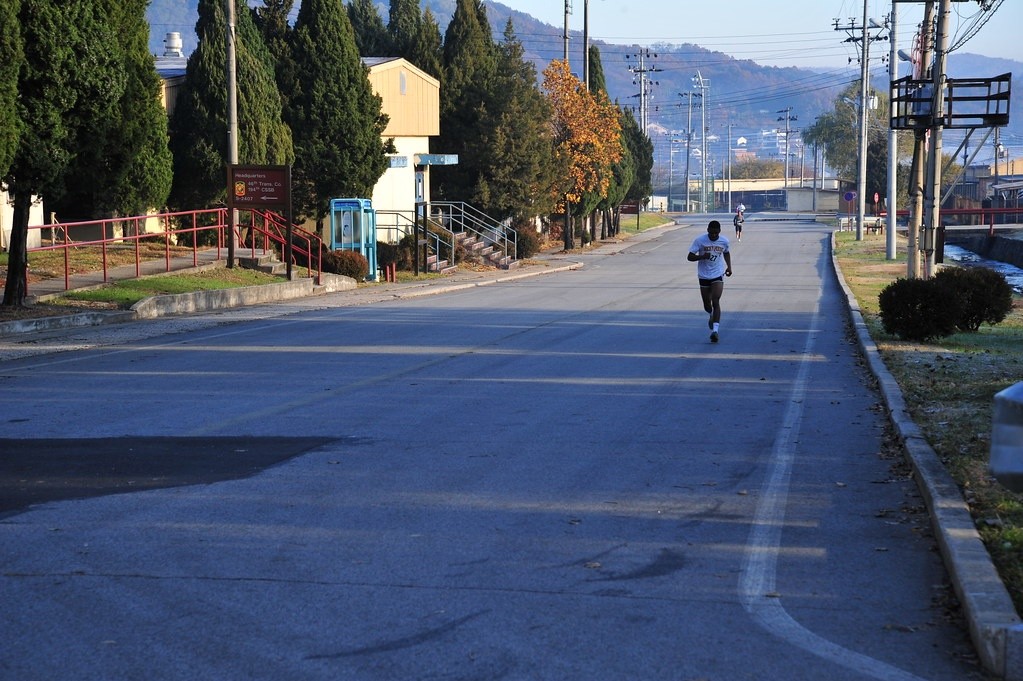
[708,315,714,329]
[736,235,738,238]
[710,331,718,343]
[738,239,740,242]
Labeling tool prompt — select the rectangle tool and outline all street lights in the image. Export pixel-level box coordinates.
[843,97,862,237]
[898,48,926,280]
[691,74,705,214]
[870,17,896,260]
[994,143,1002,199]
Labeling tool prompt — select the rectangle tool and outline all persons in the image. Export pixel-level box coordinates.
[734,210,744,242]
[999,142,1004,156]
[687,220,732,343]
[737,202,746,215]
